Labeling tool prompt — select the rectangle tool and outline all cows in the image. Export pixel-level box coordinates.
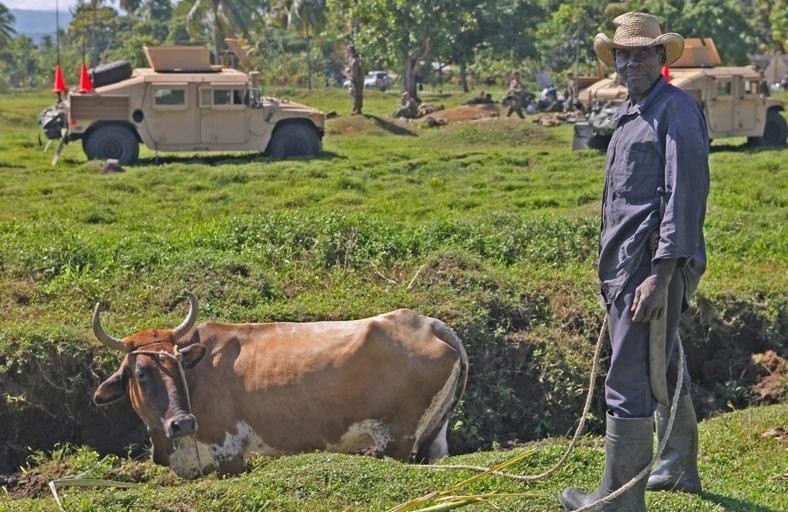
[92,288,469,479]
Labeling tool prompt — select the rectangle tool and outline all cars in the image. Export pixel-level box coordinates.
[364,70,390,91]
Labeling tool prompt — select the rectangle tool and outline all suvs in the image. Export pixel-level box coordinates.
[566,37,788,152]
[38,45,325,166]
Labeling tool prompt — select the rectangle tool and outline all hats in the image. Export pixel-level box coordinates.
[593,12,685,67]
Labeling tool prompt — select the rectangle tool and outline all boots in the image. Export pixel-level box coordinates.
[559,393,653,512]
[645,392,702,494]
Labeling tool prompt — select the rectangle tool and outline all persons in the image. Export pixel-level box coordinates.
[389,91,418,120]
[540,84,556,103]
[561,68,578,112]
[757,69,770,96]
[557,11,711,512]
[462,91,496,105]
[506,70,525,120]
[343,46,364,116]
